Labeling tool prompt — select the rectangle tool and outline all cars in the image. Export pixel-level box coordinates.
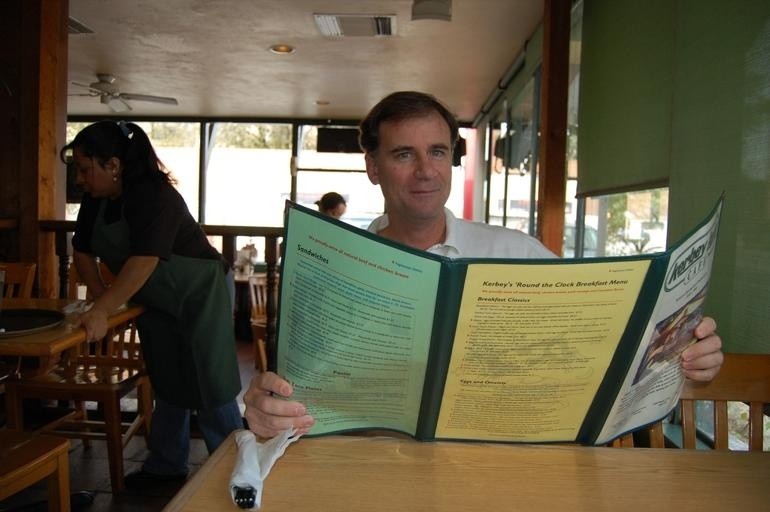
[564,226,598,258]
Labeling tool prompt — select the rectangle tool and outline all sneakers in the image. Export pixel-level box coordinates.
[125,468,186,487]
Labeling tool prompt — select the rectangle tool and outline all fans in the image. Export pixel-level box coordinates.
[66,73,177,114]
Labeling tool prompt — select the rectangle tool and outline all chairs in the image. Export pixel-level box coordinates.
[622,354,769,453]
[1,263,281,511]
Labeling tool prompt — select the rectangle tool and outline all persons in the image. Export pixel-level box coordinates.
[242,91,727,442]
[63,117,251,491]
[312,192,347,220]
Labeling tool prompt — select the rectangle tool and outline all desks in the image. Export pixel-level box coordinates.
[163,428,768,511]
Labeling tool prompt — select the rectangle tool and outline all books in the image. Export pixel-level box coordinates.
[270,191,723,444]
